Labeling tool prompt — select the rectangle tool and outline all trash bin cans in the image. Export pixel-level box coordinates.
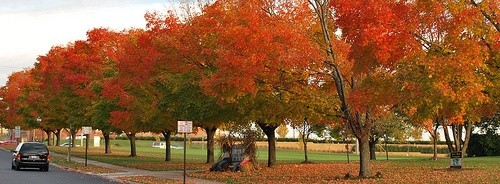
[450,151,462,169]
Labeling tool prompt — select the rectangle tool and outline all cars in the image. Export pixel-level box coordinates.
[11,141,50,173]
